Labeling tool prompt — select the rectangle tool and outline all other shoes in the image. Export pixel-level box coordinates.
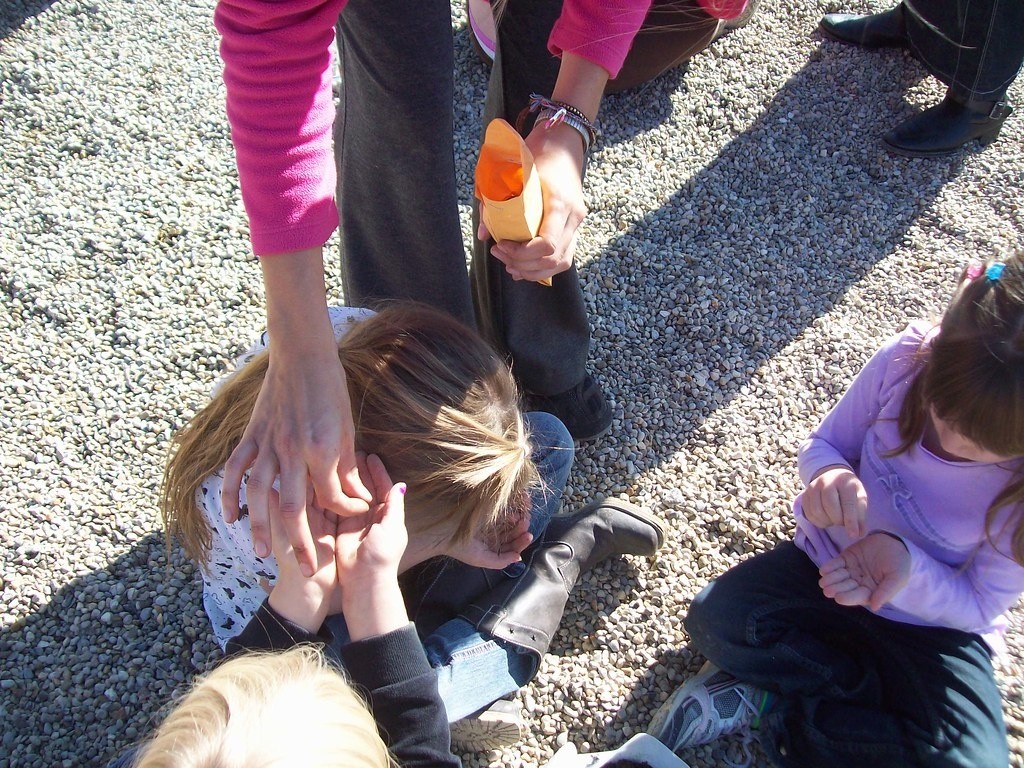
[533,370,613,444]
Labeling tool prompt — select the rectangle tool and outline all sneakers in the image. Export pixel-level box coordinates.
[645,662,777,752]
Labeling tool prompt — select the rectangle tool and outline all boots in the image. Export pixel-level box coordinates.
[415,554,502,643]
[459,497,666,672]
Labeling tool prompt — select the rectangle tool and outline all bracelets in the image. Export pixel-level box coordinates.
[516,91,597,154]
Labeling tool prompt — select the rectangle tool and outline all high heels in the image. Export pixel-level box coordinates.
[821,5,906,47]
[880,87,1012,159]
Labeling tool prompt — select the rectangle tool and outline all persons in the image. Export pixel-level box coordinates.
[213,0,652,578]
[820,0,1024,156]
[646,250,1024,768]
[466,1,763,94]
[168,304,665,722]
[110,450,463,768]
